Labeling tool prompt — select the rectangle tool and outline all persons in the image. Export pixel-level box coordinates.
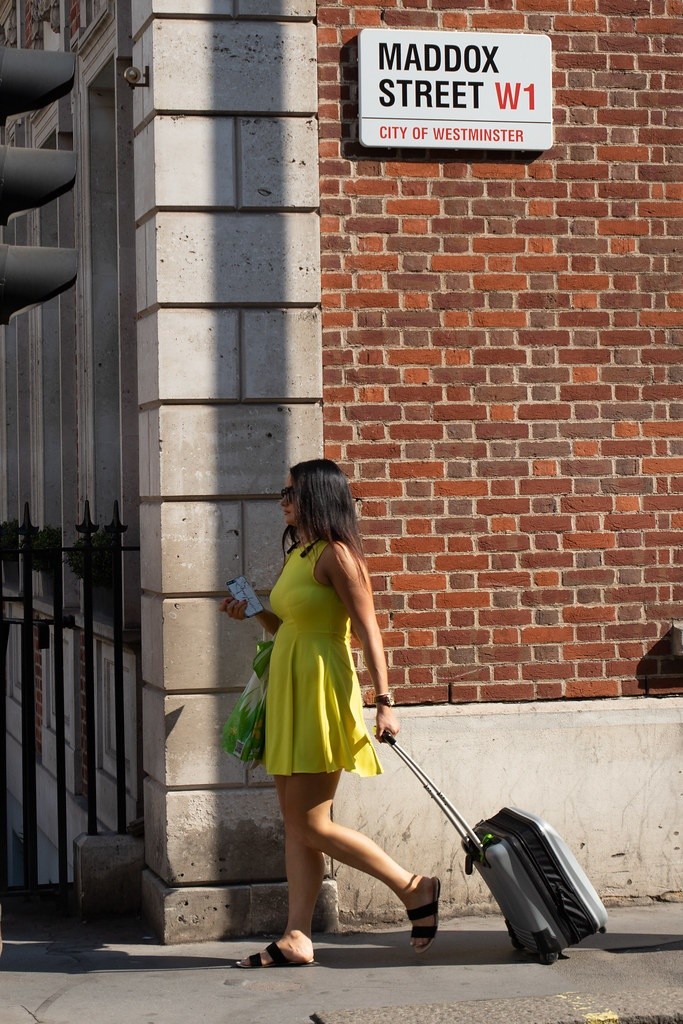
[220,458,441,971]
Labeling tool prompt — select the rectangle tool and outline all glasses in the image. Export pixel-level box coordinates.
[281,487,294,505]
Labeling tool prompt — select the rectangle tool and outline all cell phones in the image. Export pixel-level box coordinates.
[225,576,268,619]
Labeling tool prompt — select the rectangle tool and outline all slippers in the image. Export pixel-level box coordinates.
[406,876,440,953]
[235,942,321,967]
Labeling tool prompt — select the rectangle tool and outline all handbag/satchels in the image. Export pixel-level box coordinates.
[218,617,282,762]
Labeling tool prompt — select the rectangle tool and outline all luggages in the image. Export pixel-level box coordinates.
[373,726,609,964]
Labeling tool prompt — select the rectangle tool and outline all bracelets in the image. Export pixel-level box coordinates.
[375,692,391,697]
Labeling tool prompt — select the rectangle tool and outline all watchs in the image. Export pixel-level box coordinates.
[376,695,395,707]
